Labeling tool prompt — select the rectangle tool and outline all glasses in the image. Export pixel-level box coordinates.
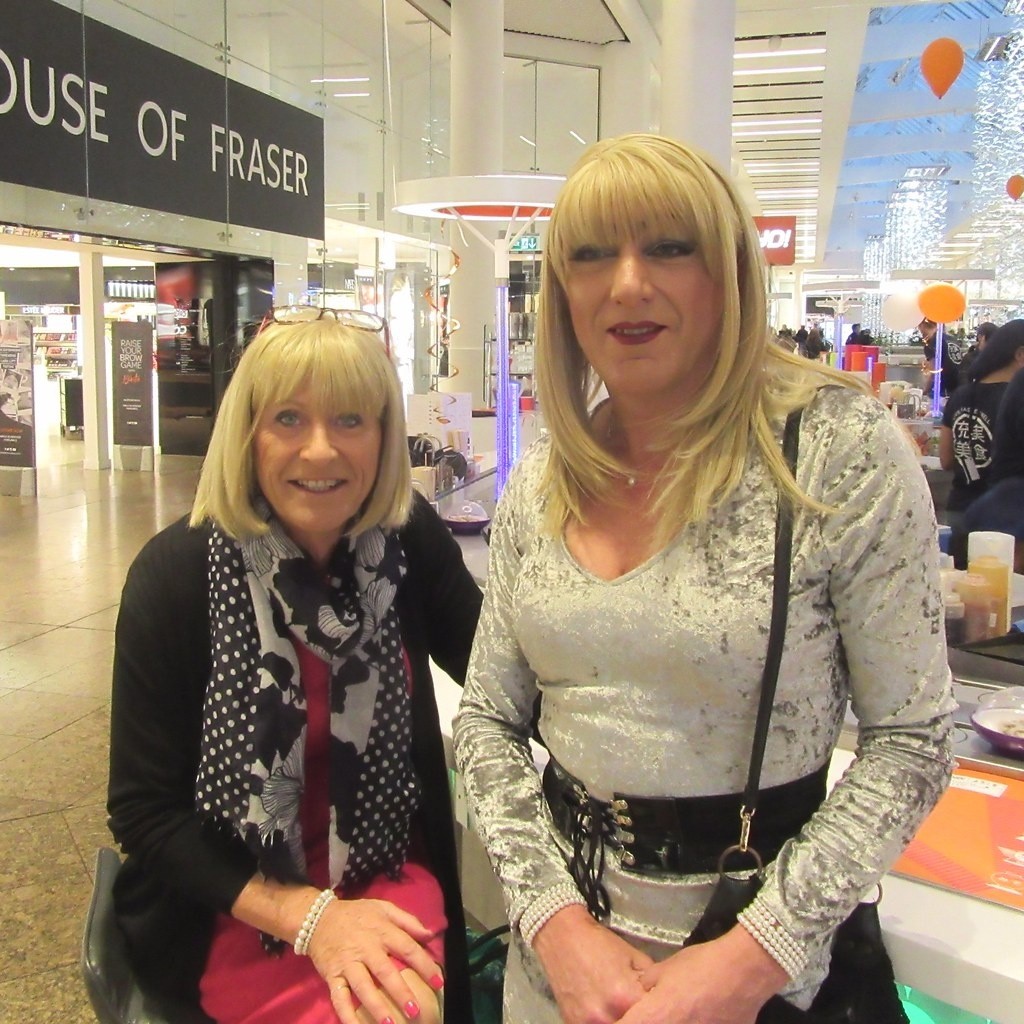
[255,305,390,361]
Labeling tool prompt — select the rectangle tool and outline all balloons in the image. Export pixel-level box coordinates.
[914,283,965,322]
[1006,175,1024,201]
[920,38,963,100]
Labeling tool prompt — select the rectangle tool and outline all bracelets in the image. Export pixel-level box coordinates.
[293,889,337,956]
[737,898,808,983]
[519,881,588,948]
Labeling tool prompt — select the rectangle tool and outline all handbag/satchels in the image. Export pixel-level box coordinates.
[683,846,910,1024]
[199,861,443,1023]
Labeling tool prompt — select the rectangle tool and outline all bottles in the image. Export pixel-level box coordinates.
[943,556,1008,646]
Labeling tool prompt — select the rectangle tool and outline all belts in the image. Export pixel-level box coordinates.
[542,754,832,875]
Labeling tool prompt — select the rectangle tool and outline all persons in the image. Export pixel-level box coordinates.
[918,318,949,397]
[939,315,1024,574]
[448,129,959,1024]
[92,304,545,1024]
[845,325,874,346]
[778,322,829,361]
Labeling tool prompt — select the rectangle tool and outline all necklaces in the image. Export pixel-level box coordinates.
[603,415,662,485]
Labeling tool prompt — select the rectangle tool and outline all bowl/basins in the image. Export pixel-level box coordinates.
[970,707,1024,757]
[444,515,491,534]
